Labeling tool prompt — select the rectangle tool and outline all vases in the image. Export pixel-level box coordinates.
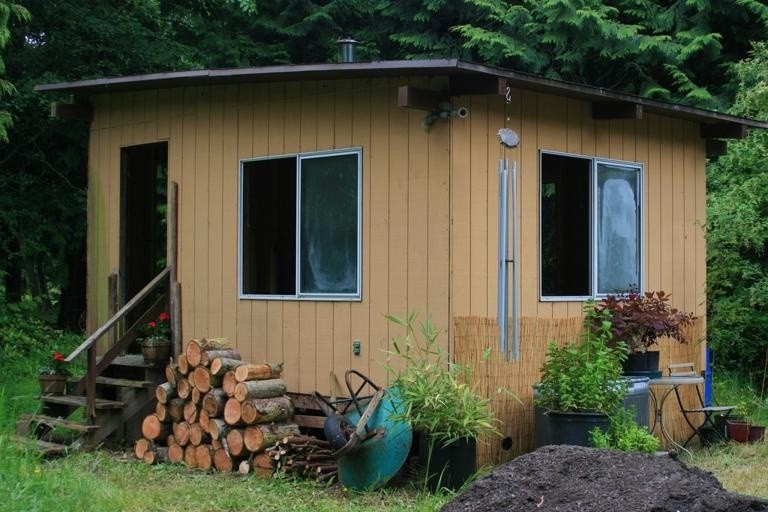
[38,375,67,396]
[141,341,171,363]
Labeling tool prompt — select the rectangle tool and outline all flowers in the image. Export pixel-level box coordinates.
[144,312,170,341]
[39,351,74,377]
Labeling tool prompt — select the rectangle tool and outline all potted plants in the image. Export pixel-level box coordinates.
[584,282,698,371]
[377,306,525,496]
[712,378,768,444]
[532,296,634,447]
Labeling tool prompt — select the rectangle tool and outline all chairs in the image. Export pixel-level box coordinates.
[667,362,737,457]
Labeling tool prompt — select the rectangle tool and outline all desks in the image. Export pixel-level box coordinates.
[649,375,705,460]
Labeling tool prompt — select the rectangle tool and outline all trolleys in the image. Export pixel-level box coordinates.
[309,369,413,494]
